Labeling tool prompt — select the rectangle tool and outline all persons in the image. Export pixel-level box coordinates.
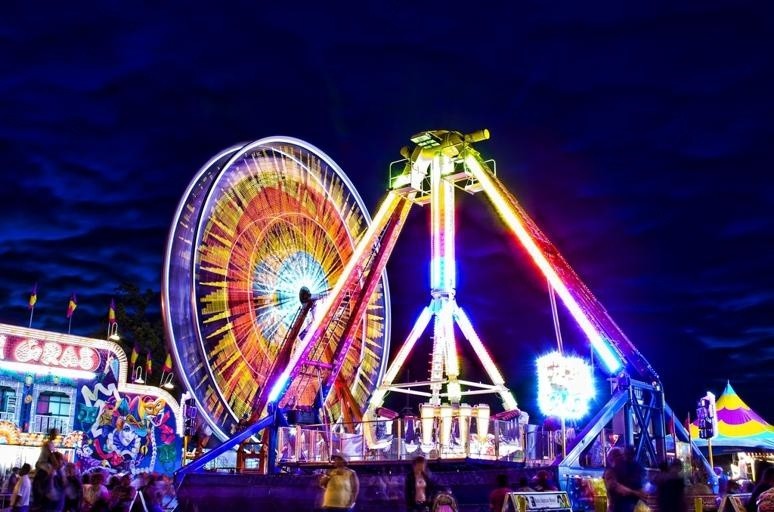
[488,473,515,511]
[404,458,452,512]
[513,477,537,493]
[0,429,184,511]
[651,459,690,511]
[615,444,648,511]
[318,451,360,512]
[603,447,657,511]
[745,460,774,511]
[531,468,559,492]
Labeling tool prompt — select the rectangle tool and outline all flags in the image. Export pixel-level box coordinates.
[144,350,152,375]
[65,291,76,319]
[27,284,37,312]
[129,341,139,368]
[158,351,172,372]
[107,298,115,324]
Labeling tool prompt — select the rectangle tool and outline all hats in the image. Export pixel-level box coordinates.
[331,451,350,466]
